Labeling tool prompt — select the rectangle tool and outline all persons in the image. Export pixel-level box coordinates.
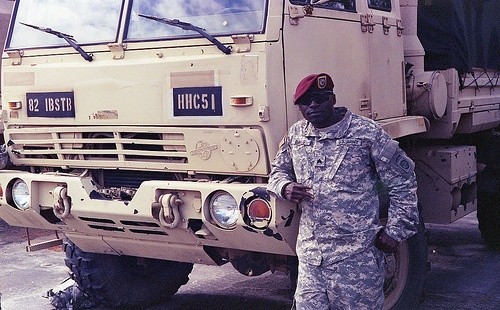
[267,71,420,310]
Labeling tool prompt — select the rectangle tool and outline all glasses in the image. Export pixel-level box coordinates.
[298,91,332,105]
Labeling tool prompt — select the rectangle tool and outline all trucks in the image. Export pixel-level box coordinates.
[1,0,500,310]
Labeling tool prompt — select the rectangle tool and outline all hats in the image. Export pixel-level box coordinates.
[292,73,334,105]
